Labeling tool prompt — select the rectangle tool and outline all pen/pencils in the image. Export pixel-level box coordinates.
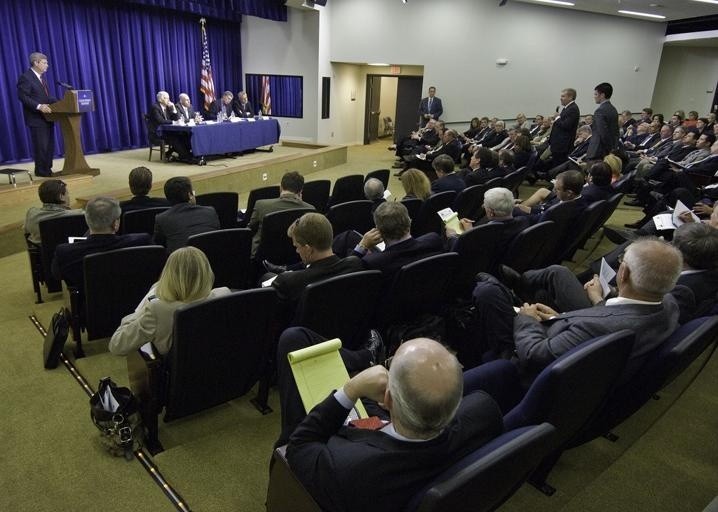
[457,220,475,223]
[677,210,694,217]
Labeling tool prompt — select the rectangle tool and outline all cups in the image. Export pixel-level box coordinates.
[194,112,200,123]
[190,119,194,125]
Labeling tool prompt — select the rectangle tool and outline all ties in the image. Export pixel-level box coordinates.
[428,99,432,111]
[40,76,48,97]
[348,415,392,431]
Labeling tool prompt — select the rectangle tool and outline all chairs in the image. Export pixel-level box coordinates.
[26,157,718,512]
[141,111,174,163]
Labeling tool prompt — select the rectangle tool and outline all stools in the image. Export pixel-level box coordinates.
[1,168,34,189]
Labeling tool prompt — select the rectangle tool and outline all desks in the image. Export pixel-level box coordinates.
[165,116,282,165]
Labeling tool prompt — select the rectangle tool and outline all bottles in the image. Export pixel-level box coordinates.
[231,111,235,121]
[217,112,220,122]
[258,110,262,119]
[180,115,184,124]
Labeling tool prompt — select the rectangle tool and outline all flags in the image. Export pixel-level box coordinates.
[200,25,217,111]
[259,75,274,116]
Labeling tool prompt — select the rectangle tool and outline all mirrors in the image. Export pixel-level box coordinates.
[243,73,304,119]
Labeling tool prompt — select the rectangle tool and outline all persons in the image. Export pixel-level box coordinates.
[23,166,233,366]
[473,235,685,374]
[419,87,443,130]
[16,52,61,178]
[547,89,580,188]
[275,327,506,512]
[392,113,592,180]
[400,150,616,250]
[234,91,255,116]
[247,171,446,289]
[619,107,718,269]
[149,91,181,159]
[173,92,205,124]
[585,82,620,171]
[208,90,235,122]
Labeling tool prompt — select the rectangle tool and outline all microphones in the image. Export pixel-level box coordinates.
[259,100,268,109]
[556,106,560,112]
[235,101,241,110]
[57,80,73,90]
[175,105,184,114]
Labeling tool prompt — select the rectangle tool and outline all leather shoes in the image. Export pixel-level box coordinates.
[624,197,643,206]
[360,328,384,367]
[263,259,286,274]
[625,214,650,229]
[473,272,500,284]
[499,263,521,289]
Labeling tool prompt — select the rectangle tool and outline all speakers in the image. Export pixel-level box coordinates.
[311,0,327,7]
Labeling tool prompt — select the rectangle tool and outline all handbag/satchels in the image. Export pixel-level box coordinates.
[89,376,146,461]
[43,305,72,369]
